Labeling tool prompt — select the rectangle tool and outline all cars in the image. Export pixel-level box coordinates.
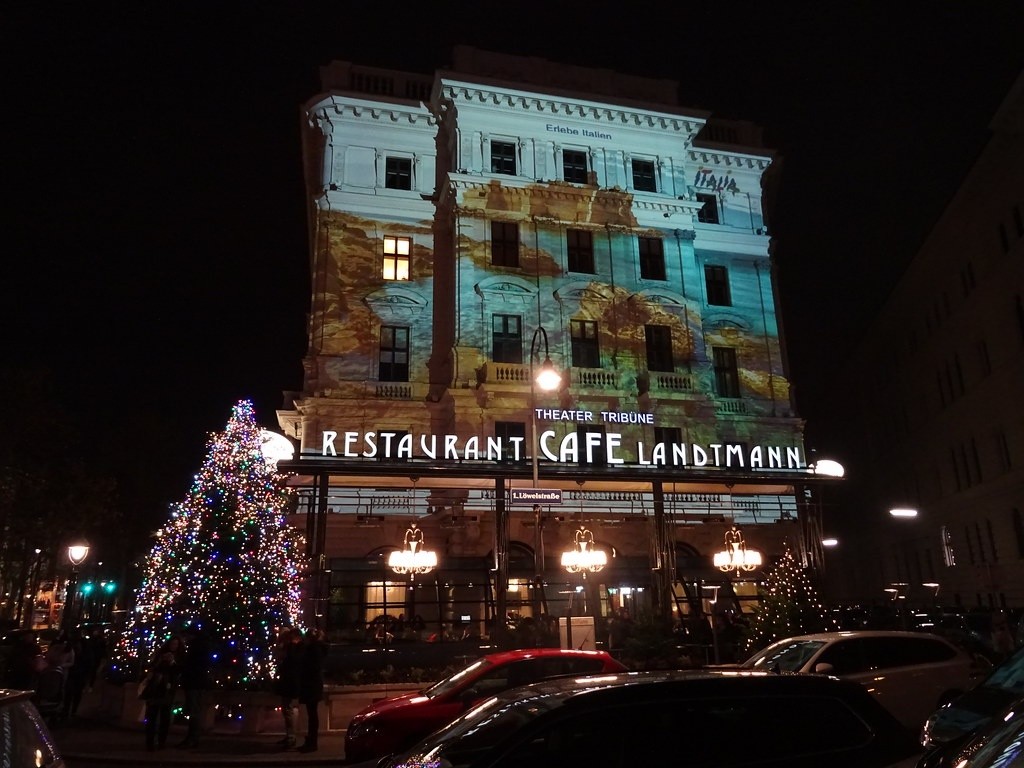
[342,648,637,764]
[741,630,990,753]
[912,651,1024,768]
[371,669,926,768]
[1,688,65,767]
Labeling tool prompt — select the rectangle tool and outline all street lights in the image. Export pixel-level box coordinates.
[59,537,92,639]
[527,326,562,615]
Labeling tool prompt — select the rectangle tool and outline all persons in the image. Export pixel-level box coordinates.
[138,634,182,750]
[5,629,117,727]
[178,633,218,750]
[290,629,329,753]
[276,641,299,750]
[508,613,523,628]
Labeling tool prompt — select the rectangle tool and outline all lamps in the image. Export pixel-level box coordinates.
[388,475,438,574]
[559,480,607,573]
[713,482,763,577]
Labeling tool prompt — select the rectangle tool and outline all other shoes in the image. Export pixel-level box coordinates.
[175,738,199,750]
[296,744,318,753]
[145,744,155,752]
[277,736,297,750]
[158,742,166,750]
